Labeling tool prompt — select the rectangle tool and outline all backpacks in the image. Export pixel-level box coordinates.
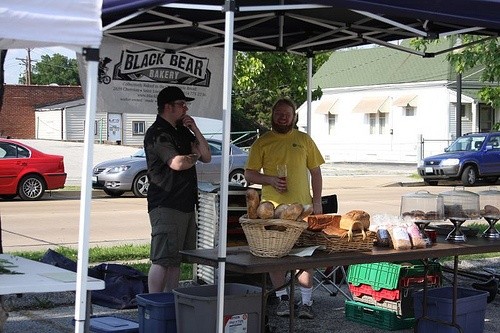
[89,263,148,309]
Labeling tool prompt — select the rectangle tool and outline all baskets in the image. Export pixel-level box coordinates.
[295,229,378,253]
[238,213,309,258]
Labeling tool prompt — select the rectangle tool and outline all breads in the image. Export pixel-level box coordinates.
[376,229,390,244]
[304,210,370,235]
[444,204,500,218]
[402,210,443,221]
[390,221,426,249]
[245,189,314,232]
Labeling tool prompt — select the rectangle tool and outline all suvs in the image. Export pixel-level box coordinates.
[417,132,500,186]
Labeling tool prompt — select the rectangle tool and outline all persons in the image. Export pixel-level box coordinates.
[144,86,212,294]
[245,98,326,319]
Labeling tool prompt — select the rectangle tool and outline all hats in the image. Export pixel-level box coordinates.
[157,86,195,106]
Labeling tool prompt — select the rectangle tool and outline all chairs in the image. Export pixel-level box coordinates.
[302,192,353,304]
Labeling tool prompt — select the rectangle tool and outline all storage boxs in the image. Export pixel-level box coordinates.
[135,291,176,333]
[342,261,489,333]
[172,281,270,333]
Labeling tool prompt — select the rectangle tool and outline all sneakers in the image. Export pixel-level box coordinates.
[297,303,315,319]
[275,294,291,316]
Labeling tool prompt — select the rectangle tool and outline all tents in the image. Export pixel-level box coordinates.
[0,0,103,333]
[99,0,500,333]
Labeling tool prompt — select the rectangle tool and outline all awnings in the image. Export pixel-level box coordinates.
[352,96,390,114]
[315,97,338,115]
[392,94,417,106]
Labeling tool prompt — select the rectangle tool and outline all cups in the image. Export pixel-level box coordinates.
[423,229,437,242]
[276,163,288,193]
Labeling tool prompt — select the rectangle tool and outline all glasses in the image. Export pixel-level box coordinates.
[174,102,186,107]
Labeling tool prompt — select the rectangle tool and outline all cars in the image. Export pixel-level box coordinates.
[0,138,67,201]
[92,138,254,197]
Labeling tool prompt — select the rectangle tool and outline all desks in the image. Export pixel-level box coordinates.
[175,234,500,333]
[0,250,106,333]
